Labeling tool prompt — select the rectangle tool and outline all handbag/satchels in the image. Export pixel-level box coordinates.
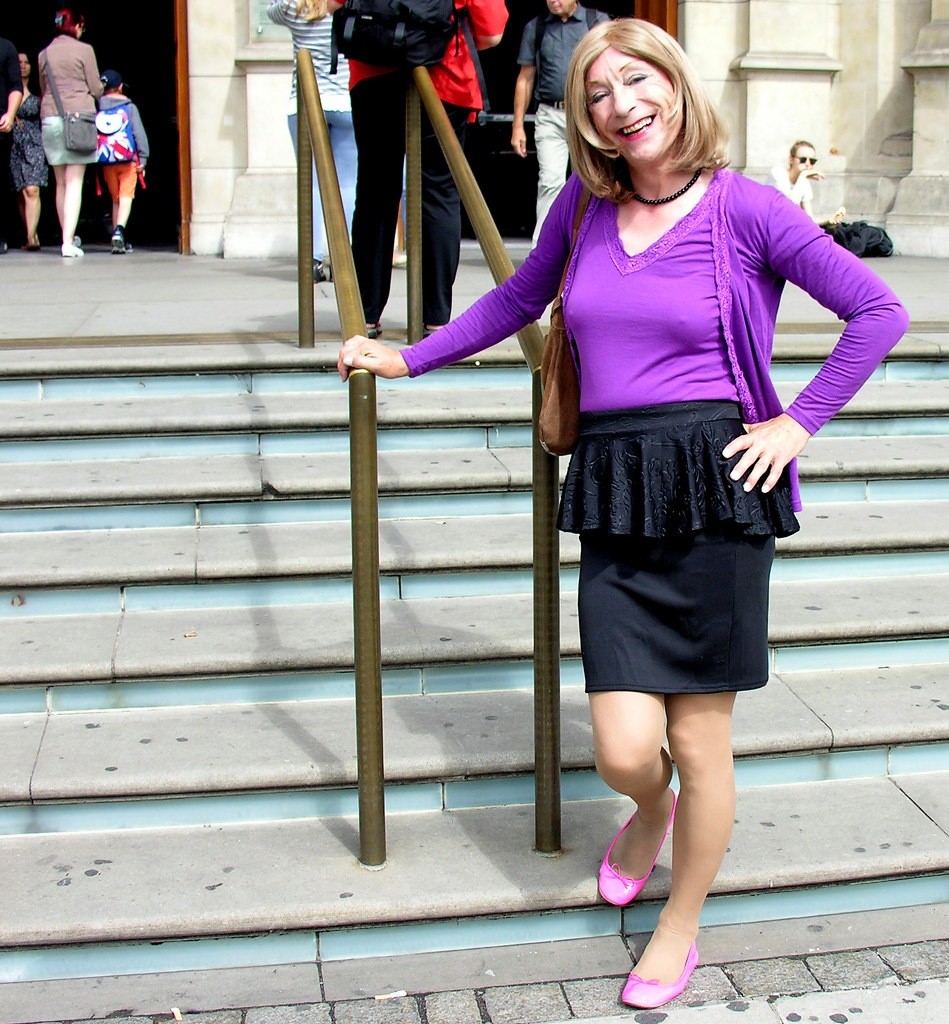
[821,222,892,257]
[64,111,98,152]
[537,183,592,456]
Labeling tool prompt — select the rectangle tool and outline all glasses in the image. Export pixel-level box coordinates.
[77,26,86,31]
[792,156,818,165]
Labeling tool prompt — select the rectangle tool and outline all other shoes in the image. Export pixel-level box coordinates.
[72,238,82,247]
[27,235,40,251]
[622,939,700,1007]
[600,785,679,904]
[61,244,85,256]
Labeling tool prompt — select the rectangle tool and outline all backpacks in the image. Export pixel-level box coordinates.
[330,0,457,71]
[93,101,135,165]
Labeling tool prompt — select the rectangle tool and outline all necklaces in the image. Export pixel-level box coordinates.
[629,170,705,205]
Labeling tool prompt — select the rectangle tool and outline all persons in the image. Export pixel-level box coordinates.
[95,71,149,254]
[769,139,848,225]
[512,0,612,247]
[336,16,909,1008]
[265,0,508,340]
[10,46,48,250]
[0,45,24,253]
[38,8,103,258]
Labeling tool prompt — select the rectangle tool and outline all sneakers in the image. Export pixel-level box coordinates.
[112,230,125,255]
[124,242,134,253]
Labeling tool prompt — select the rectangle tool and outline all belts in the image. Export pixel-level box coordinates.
[541,99,569,110]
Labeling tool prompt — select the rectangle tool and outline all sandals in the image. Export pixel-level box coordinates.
[366,321,383,339]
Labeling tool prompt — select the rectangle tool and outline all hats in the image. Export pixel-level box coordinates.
[101,69,130,90]
